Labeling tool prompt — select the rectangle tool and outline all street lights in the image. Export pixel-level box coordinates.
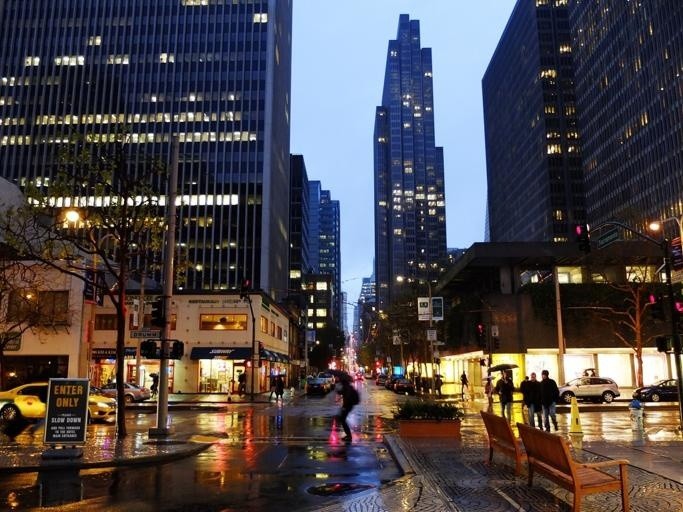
[63,207,99,384]
[648,215,681,234]
[396,275,441,400]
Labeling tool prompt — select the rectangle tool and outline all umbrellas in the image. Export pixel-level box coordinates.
[481,376,496,381]
[487,364,519,372]
[435,374,443,378]
[149,373,159,377]
[326,368,353,383]
[266,373,286,377]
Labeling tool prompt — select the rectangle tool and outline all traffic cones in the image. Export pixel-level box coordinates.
[566,395,586,434]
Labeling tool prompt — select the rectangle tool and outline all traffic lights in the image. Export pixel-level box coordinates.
[238,276,249,301]
[149,296,164,326]
[672,296,682,335]
[575,223,589,252]
[137,340,157,358]
[646,293,666,321]
[244,359,253,369]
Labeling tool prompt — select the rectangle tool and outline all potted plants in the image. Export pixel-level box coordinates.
[391,398,466,438]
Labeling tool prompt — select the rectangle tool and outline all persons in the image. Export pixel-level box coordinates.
[268,377,284,401]
[150,376,158,398]
[485,371,560,432]
[629,393,647,432]
[584,368,596,377]
[460,371,469,393]
[436,378,443,396]
[335,381,359,442]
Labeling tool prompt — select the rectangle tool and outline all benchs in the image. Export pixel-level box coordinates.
[516,422,633,511]
[480,409,530,480]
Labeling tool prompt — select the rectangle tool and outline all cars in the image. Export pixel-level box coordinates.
[306,369,374,399]
[89,380,150,404]
[375,373,413,393]
[631,375,678,403]
[0,366,127,380]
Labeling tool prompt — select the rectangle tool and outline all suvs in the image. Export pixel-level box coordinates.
[555,375,620,404]
